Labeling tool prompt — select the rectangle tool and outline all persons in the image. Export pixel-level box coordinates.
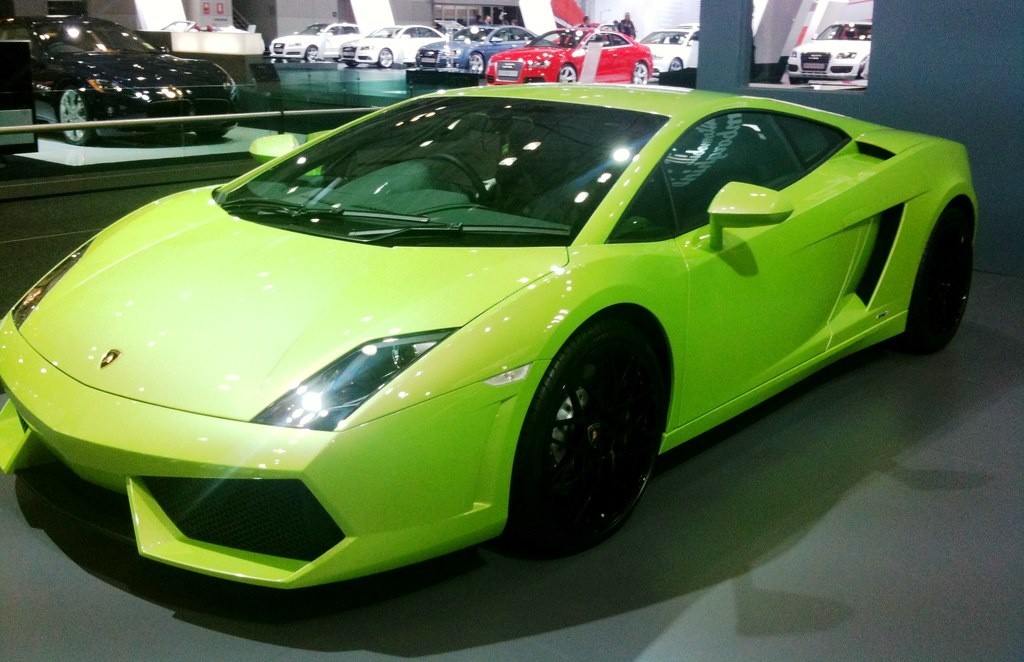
[578,16,592,27]
[613,13,636,40]
[474,15,483,24]
[483,15,493,25]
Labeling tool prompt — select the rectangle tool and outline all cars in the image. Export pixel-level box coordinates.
[337,20,700,84]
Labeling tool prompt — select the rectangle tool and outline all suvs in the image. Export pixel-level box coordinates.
[268,22,363,63]
[786,21,872,85]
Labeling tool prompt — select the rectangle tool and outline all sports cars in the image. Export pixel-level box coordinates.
[0,81,980,593]
[0,15,241,144]
